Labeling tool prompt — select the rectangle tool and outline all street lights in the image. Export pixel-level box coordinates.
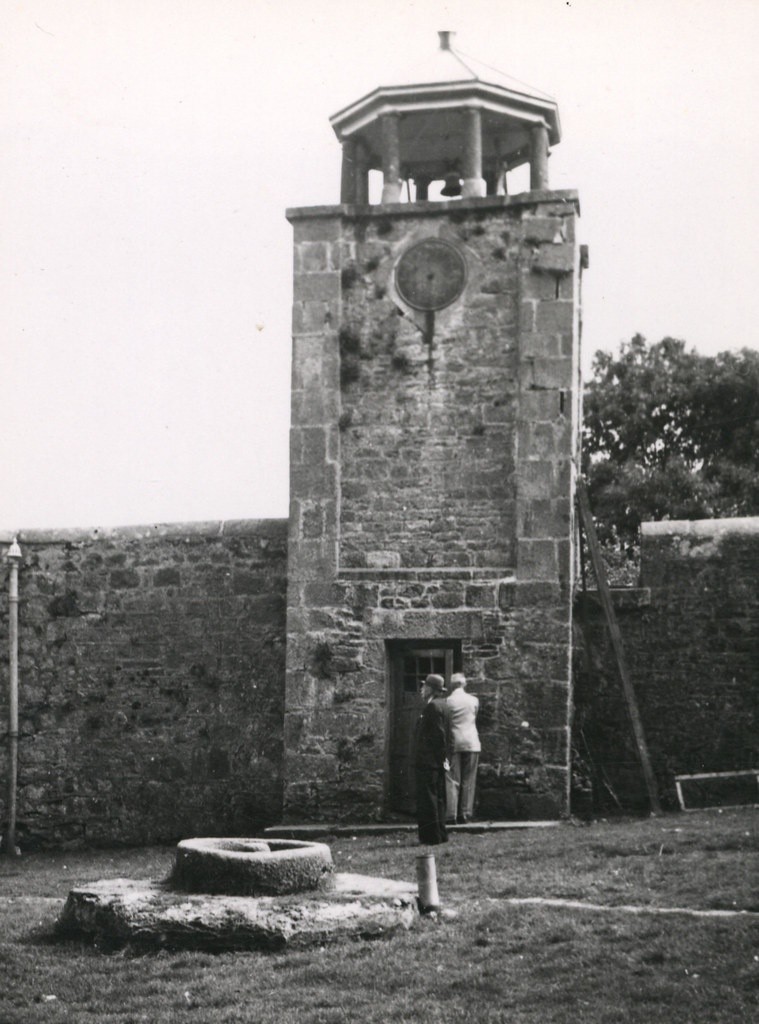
[1,537,21,858]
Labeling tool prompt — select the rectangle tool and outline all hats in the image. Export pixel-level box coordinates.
[451,673,465,683]
[421,674,447,692]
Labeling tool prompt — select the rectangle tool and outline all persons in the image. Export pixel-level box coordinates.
[445,673,480,822]
[415,674,451,844]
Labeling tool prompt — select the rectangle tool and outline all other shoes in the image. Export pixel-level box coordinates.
[411,837,448,846]
[445,817,456,824]
[457,815,467,823]
